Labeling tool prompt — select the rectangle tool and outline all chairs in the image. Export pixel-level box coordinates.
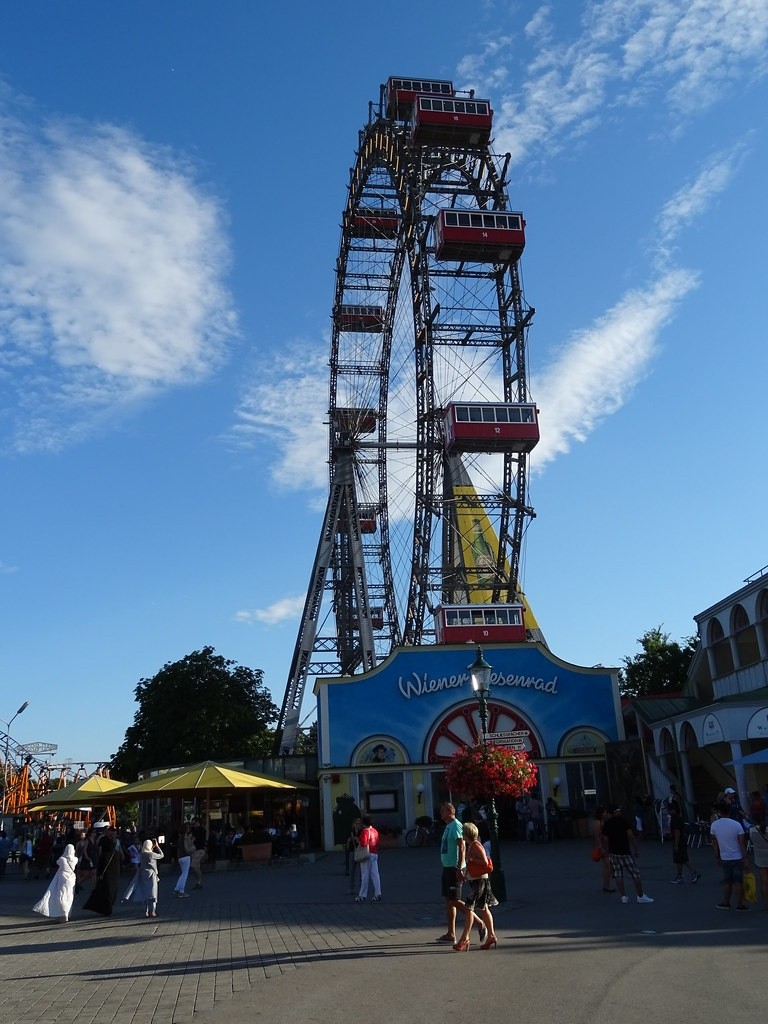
[684,821,713,849]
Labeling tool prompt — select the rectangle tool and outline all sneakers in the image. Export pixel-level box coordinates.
[621,894,629,903]
[715,902,730,909]
[636,894,654,903]
[735,904,751,912]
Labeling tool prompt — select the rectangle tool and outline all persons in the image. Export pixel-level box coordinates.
[32,844,79,923]
[21,833,33,880]
[0,831,11,875]
[592,804,654,904]
[11,834,18,864]
[373,745,387,762]
[633,796,646,841]
[130,821,137,832]
[709,787,768,912]
[128,836,164,918]
[436,800,499,952]
[515,793,561,844]
[172,816,206,898]
[75,826,125,917]
[667,784,701,883]
[348,815,382,902]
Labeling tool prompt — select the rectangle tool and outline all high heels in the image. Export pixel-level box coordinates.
[452,938,470,952]
[480,936,497,951]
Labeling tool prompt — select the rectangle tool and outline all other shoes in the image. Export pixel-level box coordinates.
[58,915,68,924]
[478,928,487,942]
[354,896,368,903]
[370,896,381,903]
[603,887,616,892]
[145,912,149,917]
[173,891,190,898]
[670,876,683,884]
[689,871,701,883]
[436,934,456,944]
[151,912,158,917]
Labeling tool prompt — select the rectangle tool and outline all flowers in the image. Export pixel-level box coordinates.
[447,742,537,797]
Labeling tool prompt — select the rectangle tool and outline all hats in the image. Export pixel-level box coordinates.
[25,832,32,838]
[725,787,736,794]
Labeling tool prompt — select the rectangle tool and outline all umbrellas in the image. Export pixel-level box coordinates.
[16,774,129,826]
[91,760,318,841]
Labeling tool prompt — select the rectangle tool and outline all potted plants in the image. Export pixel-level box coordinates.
[236,831,272,861]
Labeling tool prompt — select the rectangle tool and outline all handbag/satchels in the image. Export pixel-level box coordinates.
[354,845,370,863]
[184,833,195,855]
[741,871,758,904]
[465,843,493,876]
[591,848,600,860]
